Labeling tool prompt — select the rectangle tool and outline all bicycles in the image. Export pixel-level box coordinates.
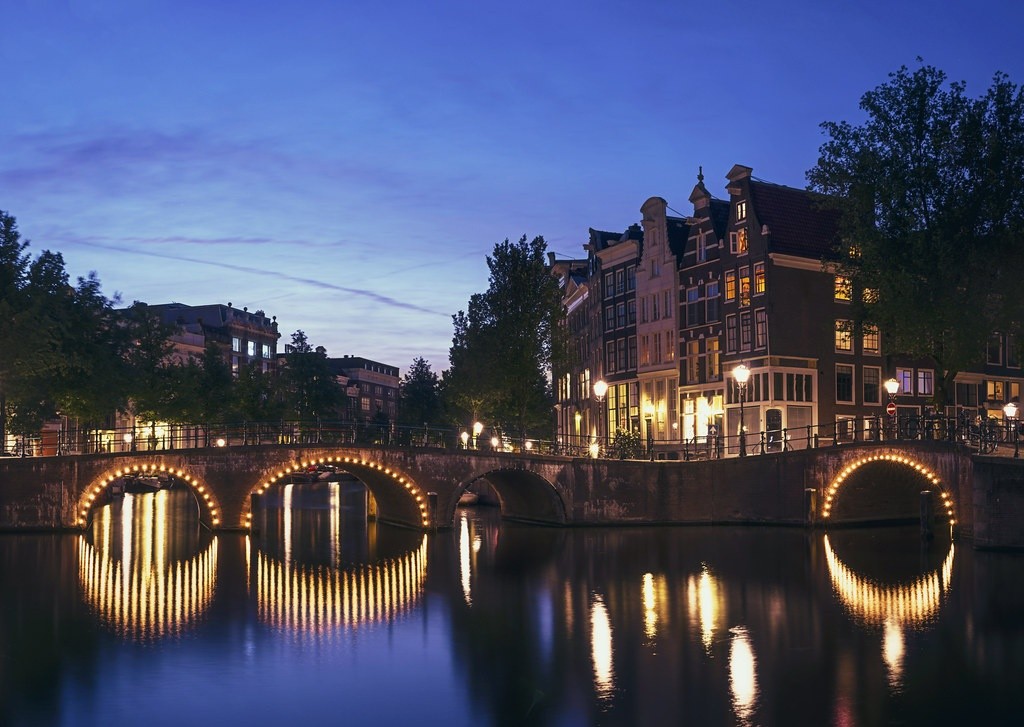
[905,406,999,454]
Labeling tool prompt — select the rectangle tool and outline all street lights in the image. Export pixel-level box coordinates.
[217,438,225,446]
[883,379,899,437]
[1002,402,1017,441]
[123,433,132,452]
[461,422,533,450]
[593,380,608,456]
[731,365,751,456]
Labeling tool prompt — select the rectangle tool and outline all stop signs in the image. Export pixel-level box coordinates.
[886,403,897,416]
